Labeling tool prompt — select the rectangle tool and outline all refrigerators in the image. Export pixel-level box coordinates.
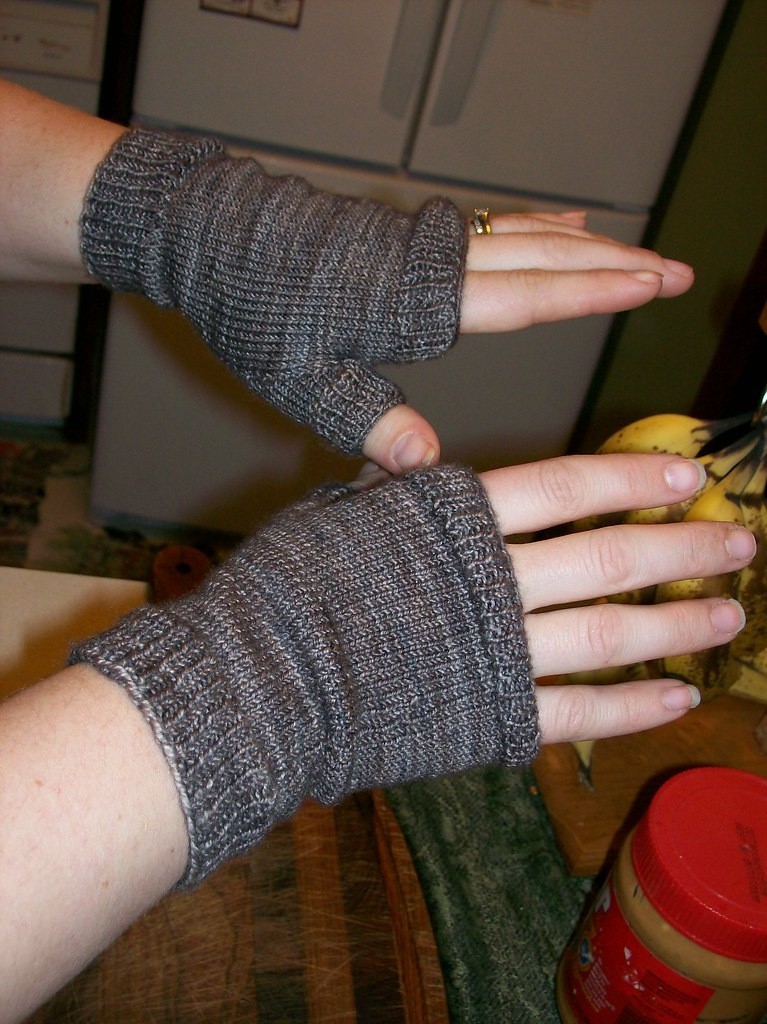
[85,0,727,545]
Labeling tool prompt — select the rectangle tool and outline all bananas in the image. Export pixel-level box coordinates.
[549,386,767,779]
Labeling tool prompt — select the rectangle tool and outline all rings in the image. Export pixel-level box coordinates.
[470,208,494,235]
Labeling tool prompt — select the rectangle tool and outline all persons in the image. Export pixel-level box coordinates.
[0,73,756,1024]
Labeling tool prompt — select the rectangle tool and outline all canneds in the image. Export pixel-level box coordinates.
[553,765,767,1024]
